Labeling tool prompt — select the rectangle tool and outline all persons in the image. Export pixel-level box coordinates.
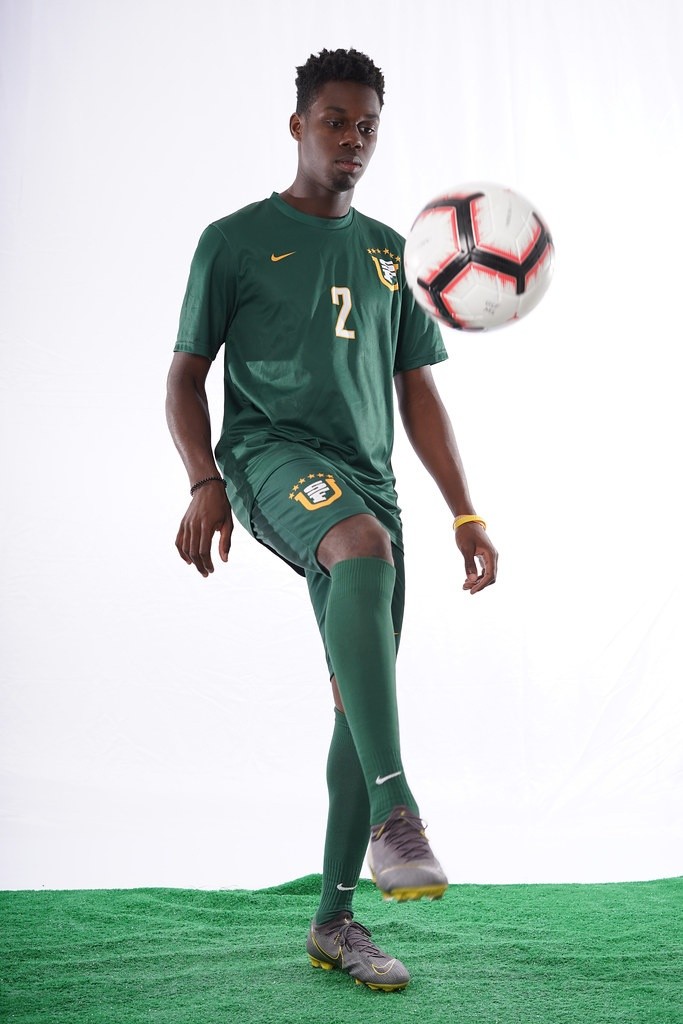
[166,41,502,993]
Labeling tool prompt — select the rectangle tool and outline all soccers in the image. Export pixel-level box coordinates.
[405,183,554,334]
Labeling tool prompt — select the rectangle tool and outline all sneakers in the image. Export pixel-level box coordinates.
[308,909,409,994]
[368,807,449,899]
